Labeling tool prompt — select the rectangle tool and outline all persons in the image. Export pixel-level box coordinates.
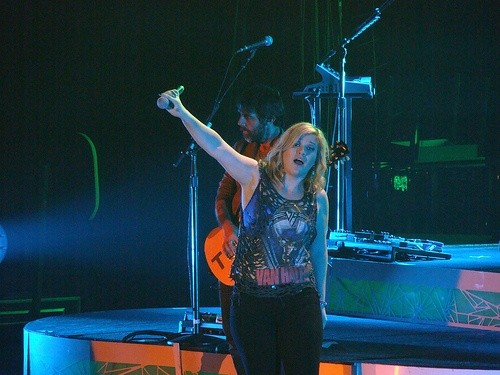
[160,89,329,375]
[215,86,286,375]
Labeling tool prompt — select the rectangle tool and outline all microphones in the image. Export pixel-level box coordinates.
[157,86,184,109]
[236,36,273,54]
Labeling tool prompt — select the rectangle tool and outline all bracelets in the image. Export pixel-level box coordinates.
[319,301,327,308]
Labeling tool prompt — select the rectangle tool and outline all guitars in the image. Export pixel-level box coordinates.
[203,141,350,286]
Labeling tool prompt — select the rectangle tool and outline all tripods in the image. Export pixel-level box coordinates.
[165,48,257,352]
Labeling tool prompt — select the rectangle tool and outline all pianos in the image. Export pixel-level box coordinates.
[294,62,374,100]
